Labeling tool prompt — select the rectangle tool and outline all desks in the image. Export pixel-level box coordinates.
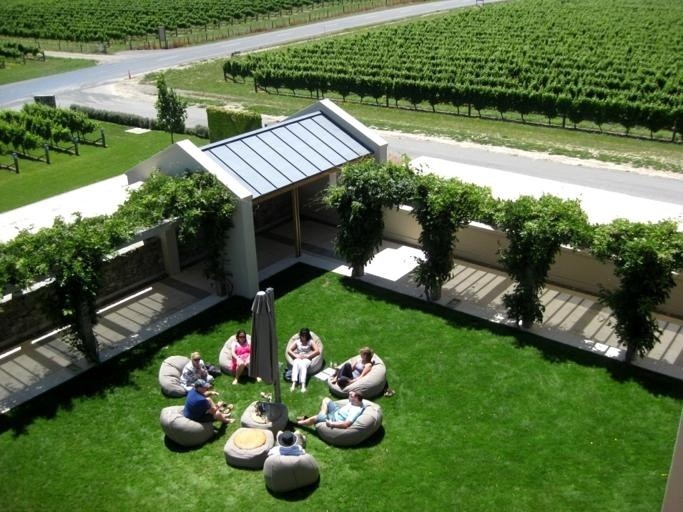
[224,401,288,469]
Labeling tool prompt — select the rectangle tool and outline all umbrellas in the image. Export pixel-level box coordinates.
[248,287,281,403]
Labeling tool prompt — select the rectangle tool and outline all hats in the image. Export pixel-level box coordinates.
[277,429,299,449]
[300,327,310,335]
[195,378,212,388]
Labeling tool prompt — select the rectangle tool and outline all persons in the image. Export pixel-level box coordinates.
[179,328,373,456]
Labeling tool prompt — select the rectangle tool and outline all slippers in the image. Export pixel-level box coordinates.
[296,415,310,421]
[219,403,234,414]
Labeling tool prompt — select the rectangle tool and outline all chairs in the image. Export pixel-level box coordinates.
[285,331,325,375]
[327,351,387,400]
[262,446,319,493]
[158,355,205,398]
[218,334,251,375]
[160,405,213,447]
[314,398,383,449]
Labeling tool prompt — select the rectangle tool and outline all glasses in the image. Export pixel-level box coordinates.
[192,357,201,361]
[239,336,245,338]
